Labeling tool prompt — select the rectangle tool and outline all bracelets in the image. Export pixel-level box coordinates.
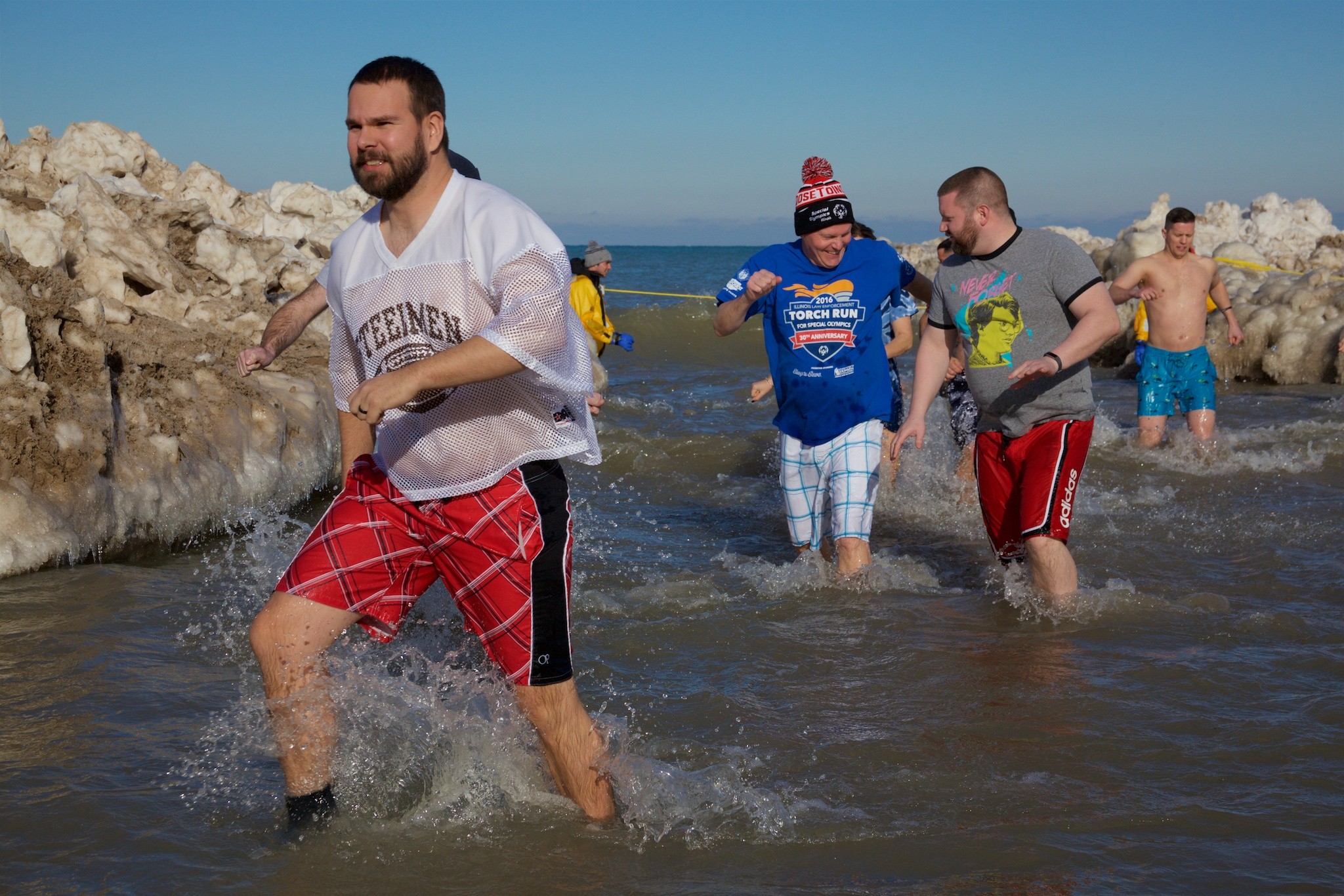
[1220,306,1233,313]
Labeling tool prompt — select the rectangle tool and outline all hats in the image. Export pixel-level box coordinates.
[794,155,855,236]
[584,239,612,268]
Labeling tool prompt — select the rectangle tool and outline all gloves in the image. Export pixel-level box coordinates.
[611,332,635,352]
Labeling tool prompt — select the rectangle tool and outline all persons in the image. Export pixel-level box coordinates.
[1109,207,1245,449]
[888,165,1123,603]
[246,56,614,837]
[234,131,604,422]
[1131,246,1221,372]
[711,156,934,580]
[747,218,921,496]
[569,236,634,418]
[907,204,1075,519]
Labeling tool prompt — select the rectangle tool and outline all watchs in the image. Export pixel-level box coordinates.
[1044,352,1062,374]
